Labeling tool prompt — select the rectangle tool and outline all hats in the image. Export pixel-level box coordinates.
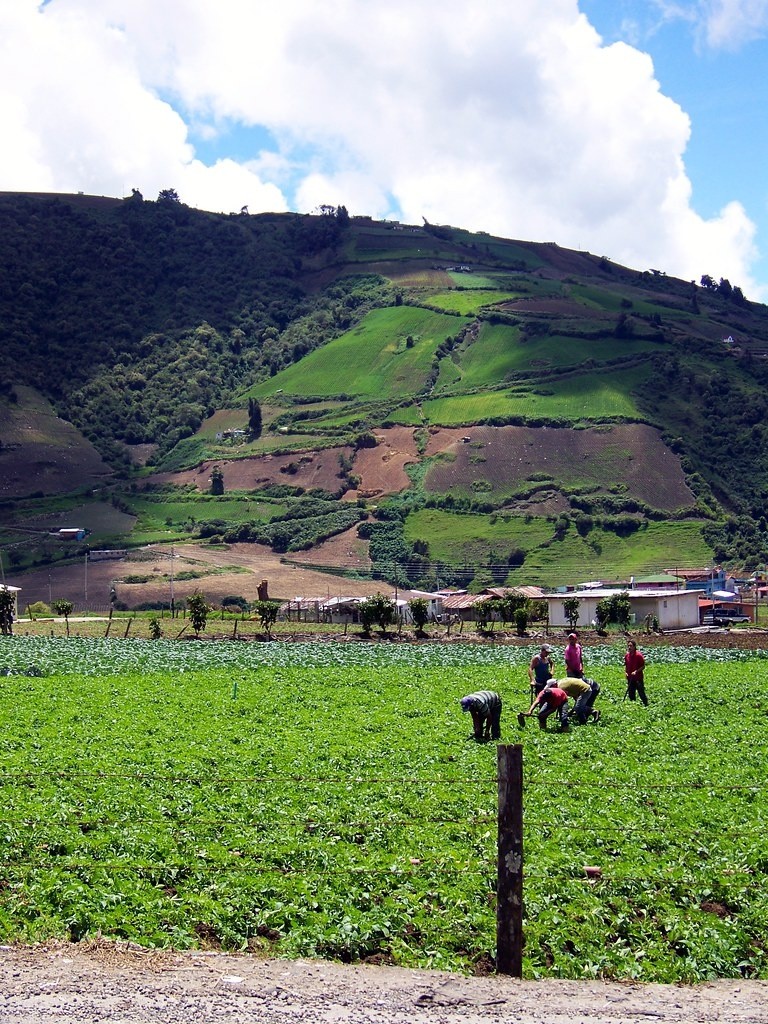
[541,643,553,653]
[567,634,577,640]
[460,697,471,712]
[541,690,552,700]
[543,679,557,689]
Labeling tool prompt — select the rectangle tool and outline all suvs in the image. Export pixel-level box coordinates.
[704,608,752,629]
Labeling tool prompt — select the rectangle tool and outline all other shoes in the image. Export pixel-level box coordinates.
[592,710,601,723]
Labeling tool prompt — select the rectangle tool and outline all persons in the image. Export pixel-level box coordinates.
[623,641,648,706]
[459,633,601,741]
